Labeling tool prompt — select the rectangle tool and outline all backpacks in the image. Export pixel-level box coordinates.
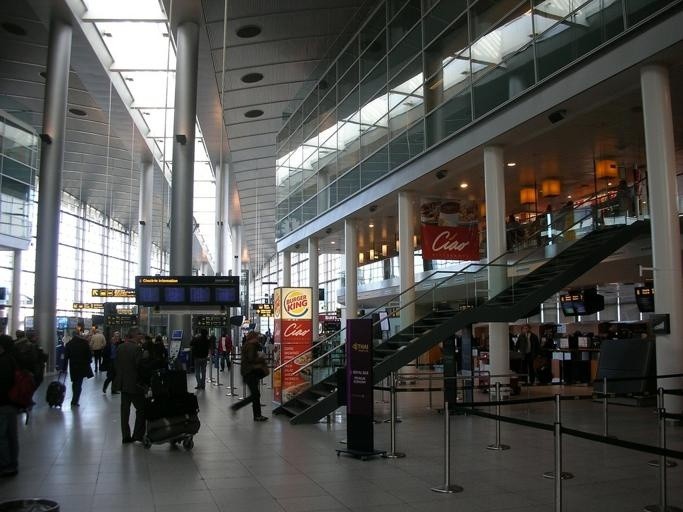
[7,335,48,412]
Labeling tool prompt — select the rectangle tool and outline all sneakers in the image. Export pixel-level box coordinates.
[254,416,268,421]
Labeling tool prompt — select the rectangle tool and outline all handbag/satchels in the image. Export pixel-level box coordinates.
[253,360,270,378]
[146,370,201,441]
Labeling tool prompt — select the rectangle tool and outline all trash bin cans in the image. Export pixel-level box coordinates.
[56,344,67,371]
[0,498,61,512]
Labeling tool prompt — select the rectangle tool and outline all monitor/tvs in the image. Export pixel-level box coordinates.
[172,330,183,339]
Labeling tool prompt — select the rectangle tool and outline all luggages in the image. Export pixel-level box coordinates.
[46,371,67,407]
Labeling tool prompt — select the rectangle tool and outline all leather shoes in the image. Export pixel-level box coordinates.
[196,385,205,390]
[71,401,80,406]
[122,436,142,443]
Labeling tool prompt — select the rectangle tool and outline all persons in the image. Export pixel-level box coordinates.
[509,325,557,386]
[231,332,269,421]
[119,328,150,443]
[13,325,272,407]
[0,334,19,477]
[616,180,634,216]
[507,200,574,248]
